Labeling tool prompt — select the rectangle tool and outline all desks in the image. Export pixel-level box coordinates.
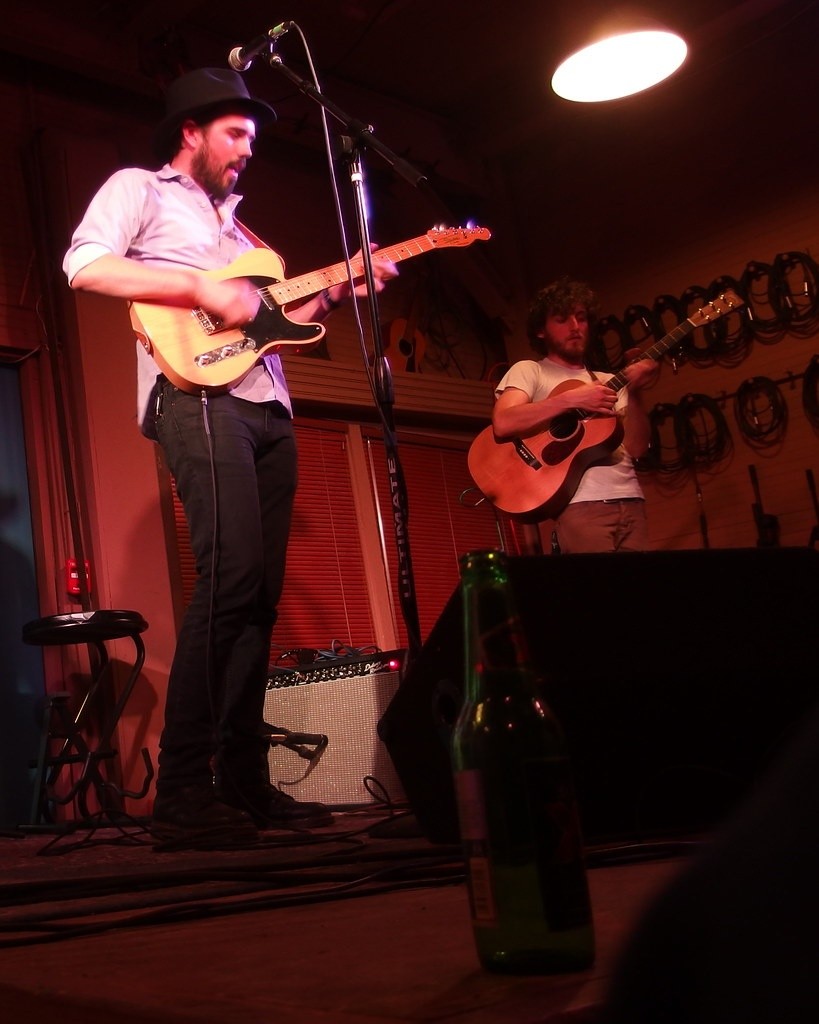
[377,547,819,845]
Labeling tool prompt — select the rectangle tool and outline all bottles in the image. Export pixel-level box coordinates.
[450,548,595,975]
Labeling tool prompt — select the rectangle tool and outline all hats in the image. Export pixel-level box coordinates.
[155,69,277,150]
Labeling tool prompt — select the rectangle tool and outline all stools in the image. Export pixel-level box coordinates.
[16,609,154,833]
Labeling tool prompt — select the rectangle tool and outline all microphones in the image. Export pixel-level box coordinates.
[265,723,328,760]
[228,21,295,72]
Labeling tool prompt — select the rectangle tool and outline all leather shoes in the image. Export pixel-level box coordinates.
[214,784,335,829]
[148,781,249,849]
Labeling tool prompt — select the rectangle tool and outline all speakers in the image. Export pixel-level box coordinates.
[267,647,408,805]
[377,543,819,857]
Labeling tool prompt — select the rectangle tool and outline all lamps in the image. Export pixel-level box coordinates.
[551,9,691,103]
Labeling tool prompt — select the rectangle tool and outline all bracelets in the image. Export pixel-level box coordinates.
[322,289,341,310]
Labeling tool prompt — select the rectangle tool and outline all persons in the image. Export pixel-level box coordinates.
[63,69,398,839]
[492,282,659,554]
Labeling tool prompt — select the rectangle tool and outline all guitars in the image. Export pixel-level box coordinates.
[466,288,749,523]
[127,219,491,394]
